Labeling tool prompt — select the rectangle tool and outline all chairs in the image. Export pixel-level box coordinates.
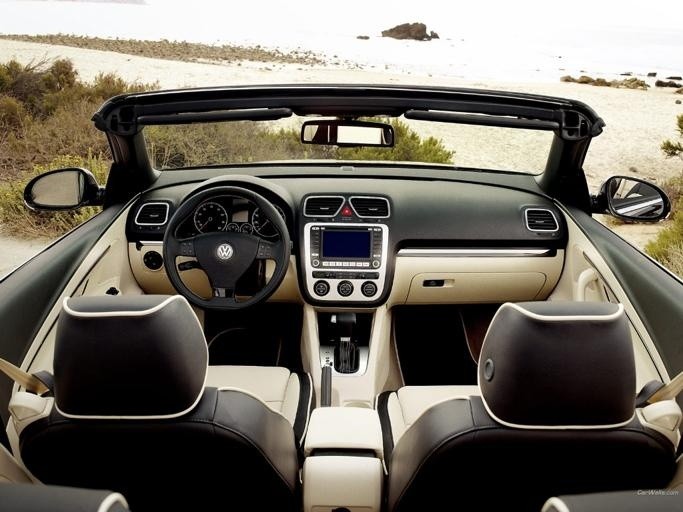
[376,296,680,512]
[10,296,315,511]
